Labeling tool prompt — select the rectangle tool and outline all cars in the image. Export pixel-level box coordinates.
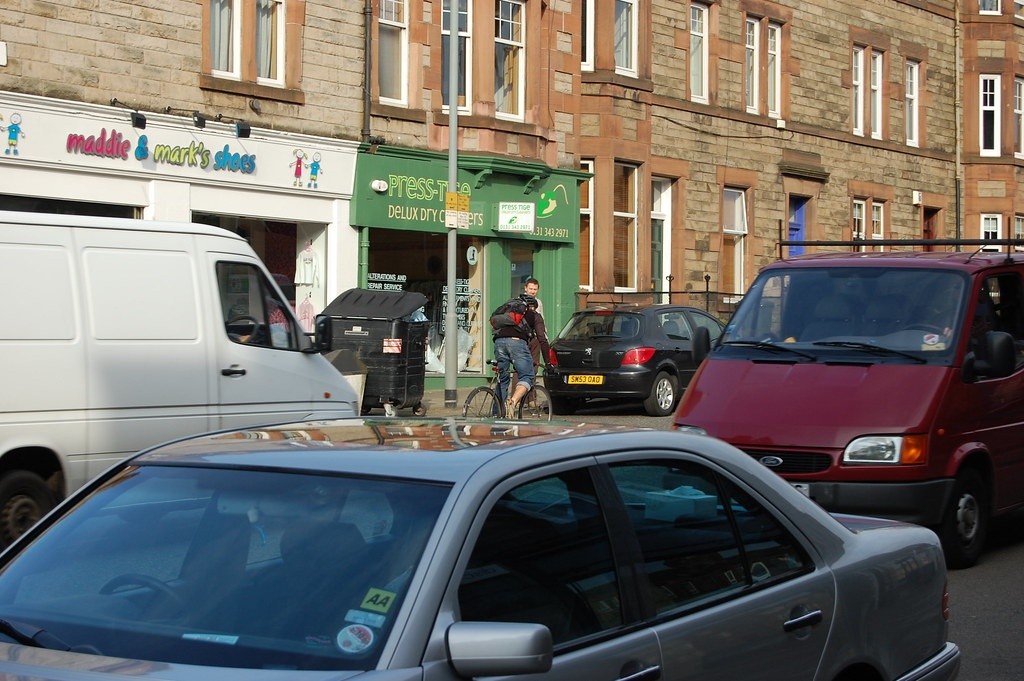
[1,415,963,681]
[542,302,742,417]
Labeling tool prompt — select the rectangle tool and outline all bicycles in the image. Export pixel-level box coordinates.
[462,359,554,423]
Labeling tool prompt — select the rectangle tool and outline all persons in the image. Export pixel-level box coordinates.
[929,275,993,348]
[227,332,251,343]
[492,279,554,420]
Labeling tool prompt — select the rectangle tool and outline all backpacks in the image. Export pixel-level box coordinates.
[490,297,533,337]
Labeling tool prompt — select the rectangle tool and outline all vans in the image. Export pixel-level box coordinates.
[1,214,362,566]
[667,251,1024,571]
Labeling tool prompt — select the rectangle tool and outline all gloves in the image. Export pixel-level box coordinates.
[543,363,554,375]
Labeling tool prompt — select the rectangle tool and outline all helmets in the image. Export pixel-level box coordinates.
[519,294,538,309]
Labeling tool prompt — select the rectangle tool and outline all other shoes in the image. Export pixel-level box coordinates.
[505,399,515,420]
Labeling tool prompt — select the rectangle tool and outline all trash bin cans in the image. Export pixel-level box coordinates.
[319,288,427,418]
